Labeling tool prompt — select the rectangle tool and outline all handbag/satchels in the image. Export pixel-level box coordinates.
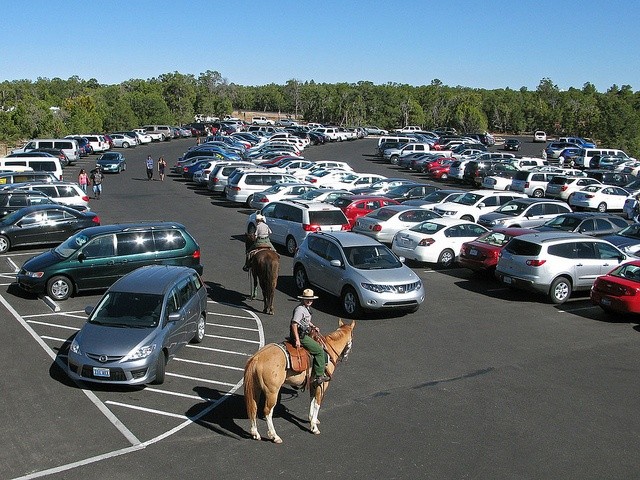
[87,177,92,185]
[164,164,166,167]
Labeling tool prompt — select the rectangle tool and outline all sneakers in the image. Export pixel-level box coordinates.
[317,375,330,384]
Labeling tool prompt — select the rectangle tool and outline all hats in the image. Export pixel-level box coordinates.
[297,289,319,300]
[256,214,265,220]
[96,164,101,168]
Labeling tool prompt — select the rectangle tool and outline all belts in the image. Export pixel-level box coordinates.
[95,183,101,185]
[258,236,269,239]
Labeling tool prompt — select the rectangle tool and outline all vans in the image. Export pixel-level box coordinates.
[0,171,59,189]
[226,169,304,208]
[0,157,63,182]
[512,169,566,197]
[20,138,79,167]
[16,222,202,301]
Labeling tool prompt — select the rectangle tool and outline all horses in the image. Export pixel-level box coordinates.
[244,231,279,315]
[244,317,357,444]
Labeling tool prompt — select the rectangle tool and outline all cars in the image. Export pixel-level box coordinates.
[536,212,628,237]
[332,194,334,195]
[402,188,465,207]
[173,163,206,184]
[333,194,401,229]
[306,167,357,185]
[369,125,512,180]
[292,229,425,317]
[458,228,540,279]
[568,183,635,211]
[586,164,640,183]
[270,160,311,174]
[391,217,491,268]
[0,190,87,220]
[7,152,55,157]
[0,204,100,254]
[384,183,442,202]
[603,223,640,257]
[297,189,355,203]
[513,130,640,164]
[96,152,127,174]
[351,204,443,244]
[28,148,69,169]
[590,260,640,314]
[246,199,351,256]
[295,160,353,177]
[351,177,416,194]
[66,125,201,151]
[208,163,269,196]
[252,182,318,210]
[321,173,388,191]
[176,113,369,163]
[67,265,208,387]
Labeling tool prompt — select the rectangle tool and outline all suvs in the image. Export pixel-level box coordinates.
[545,175,604,201]
[433,189,529,222]
[479,197,573,228]
[494,231,640,303]
[12,183,89,207]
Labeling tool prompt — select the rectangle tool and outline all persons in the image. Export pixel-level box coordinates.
[569,159,575,169]
[557,154,565,165]
[78,169,91,193]
[157,157,167,181]
[290,287,331,385]
[146,155,153,180]
[540,148,548,154]
[246,213,280,266]
[196,134,200,146]
[91,169,104,197]
